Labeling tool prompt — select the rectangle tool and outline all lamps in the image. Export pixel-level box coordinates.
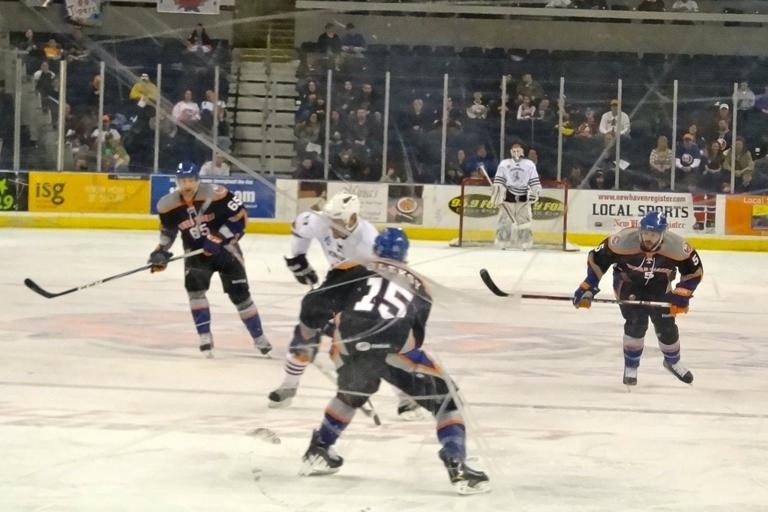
[184,21,215,54]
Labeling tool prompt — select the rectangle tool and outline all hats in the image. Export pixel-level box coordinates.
[720,103,728,110]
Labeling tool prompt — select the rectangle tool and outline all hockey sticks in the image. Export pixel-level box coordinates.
[477,162,534,229]
[24,248,203,299]
[479,268,672,309]
[303,275,379,426]
[313,358,389,417]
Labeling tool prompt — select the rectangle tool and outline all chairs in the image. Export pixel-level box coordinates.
[0,30,228,178]
[307,44,767,194]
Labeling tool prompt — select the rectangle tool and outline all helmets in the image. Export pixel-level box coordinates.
[324,192,360,231]
[176,162,200,181]
[641,212,667,232]
[510,144,524,160]
[374,227,409,260]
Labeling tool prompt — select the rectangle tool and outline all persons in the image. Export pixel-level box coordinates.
[488,142,544,249]
[293,22,401,183]
[573,213,703,387]
[147,161,273,354]
[511,1,699,23]
[298,227,489,494]
[21,19,228,172]
[267,194,433,416]
[405,69,630,190]
[649,82,756,194]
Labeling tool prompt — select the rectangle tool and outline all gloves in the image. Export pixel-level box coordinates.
[148,249,168,272]
[671,295,689,314]
[284,254,317,284]
[573,278,600,308]
[203,233,222,256]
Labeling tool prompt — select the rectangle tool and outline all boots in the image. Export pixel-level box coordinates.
[663,351,693,383]
[243,314,272,349]
[266,356,488,485]
[623,356,637,384]
[198,321,213,350]
